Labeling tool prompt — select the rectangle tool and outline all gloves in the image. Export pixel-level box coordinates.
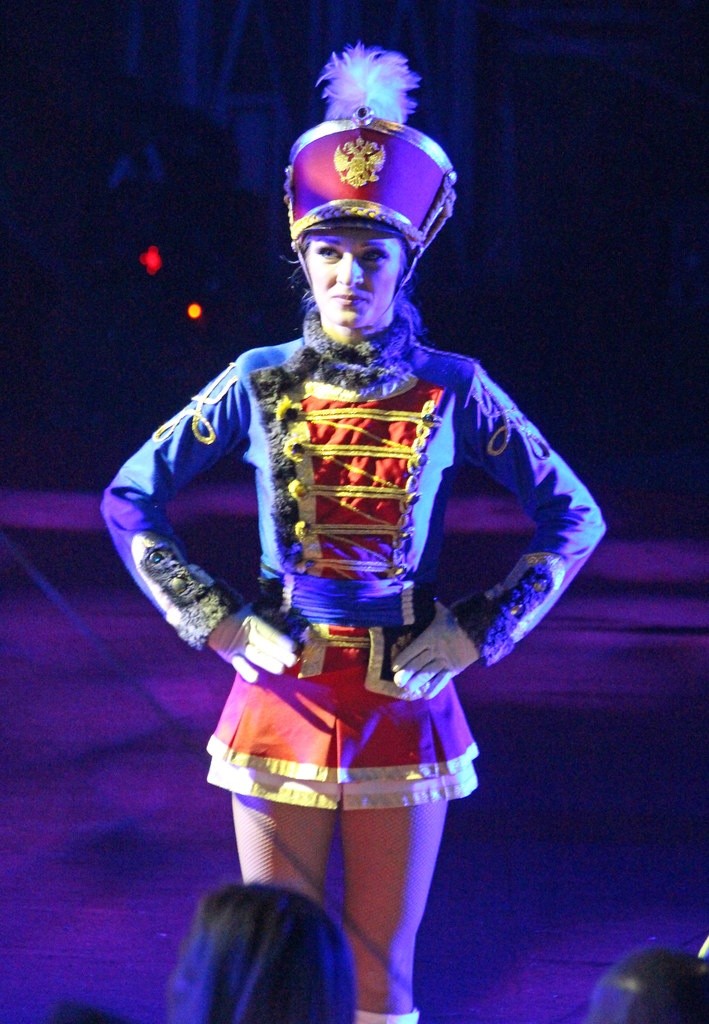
[207,601,299,684]
[390,598,483,700]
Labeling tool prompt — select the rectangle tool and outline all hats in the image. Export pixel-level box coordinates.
[283,43,458,252]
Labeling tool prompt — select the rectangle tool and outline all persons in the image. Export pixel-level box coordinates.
[168,884,358,1024]
[99,41,607,1024]
[592,948,709,1024]
